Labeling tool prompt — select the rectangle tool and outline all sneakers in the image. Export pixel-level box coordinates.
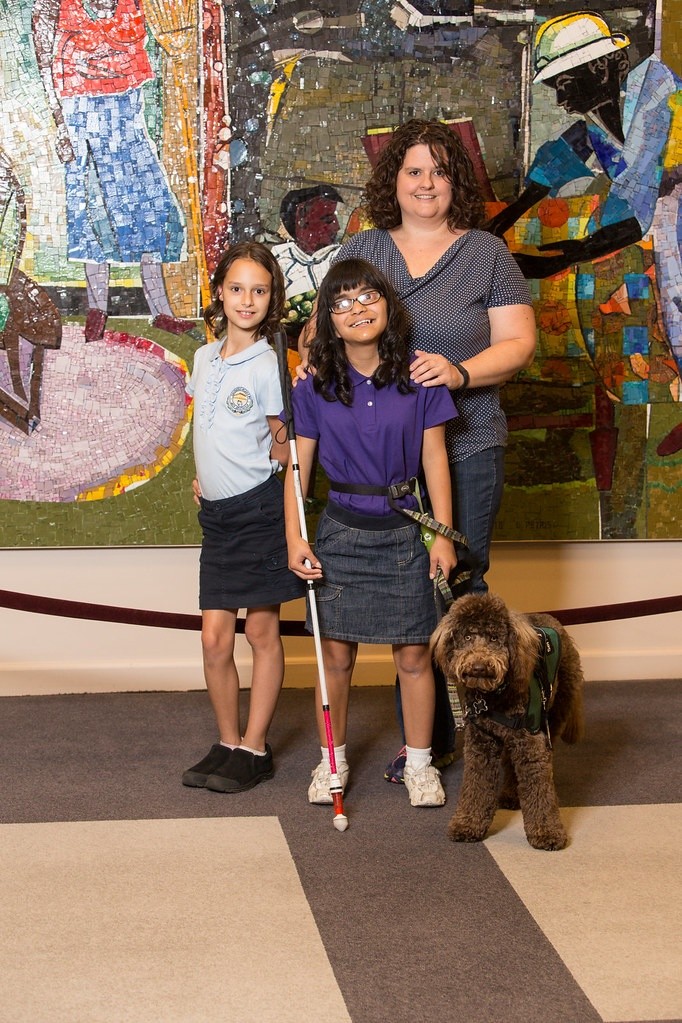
[403,761,448,807]
[308,758,350,803]
[428,752,455,769]
[383,745,407,783]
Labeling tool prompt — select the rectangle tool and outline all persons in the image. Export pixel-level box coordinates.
[181,241,298,798]
[290,119,540,785]
[282,257,460,807]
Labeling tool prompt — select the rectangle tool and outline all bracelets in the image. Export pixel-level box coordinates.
[452,361,470,391]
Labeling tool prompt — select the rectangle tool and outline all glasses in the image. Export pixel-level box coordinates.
[329,290,385,314]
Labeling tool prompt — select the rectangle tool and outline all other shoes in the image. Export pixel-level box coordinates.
[183,742,275,793]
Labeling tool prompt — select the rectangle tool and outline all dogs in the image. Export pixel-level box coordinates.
[429,592,586,851]
